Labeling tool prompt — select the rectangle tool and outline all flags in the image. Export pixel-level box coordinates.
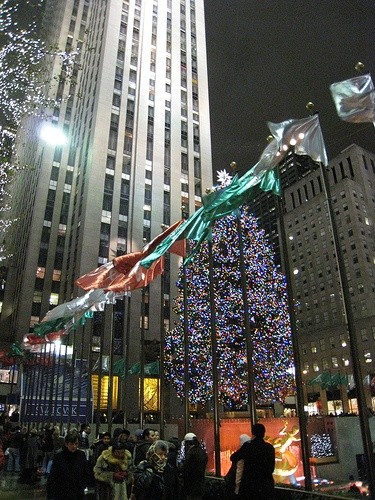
[6,115,329,362]
[91,354,160,378]
[329,73,375,123]
[310,370,348,390]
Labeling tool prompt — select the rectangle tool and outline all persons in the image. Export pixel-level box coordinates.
[224,423,275,500]
[0,406,208,500]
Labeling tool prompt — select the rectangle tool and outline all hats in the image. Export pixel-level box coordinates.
[184,433,197,440]
